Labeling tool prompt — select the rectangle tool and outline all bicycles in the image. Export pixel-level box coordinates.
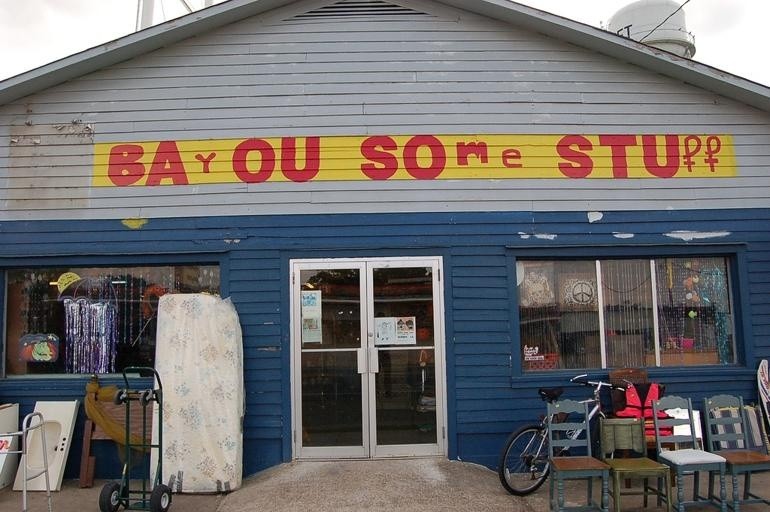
[499,372,626,496]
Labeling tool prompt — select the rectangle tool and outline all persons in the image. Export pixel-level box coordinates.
[376,313,433,401]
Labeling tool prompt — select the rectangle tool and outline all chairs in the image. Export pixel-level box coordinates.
[545,392,770,512]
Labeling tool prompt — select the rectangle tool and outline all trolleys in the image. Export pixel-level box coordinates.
[98,364,172,512]
